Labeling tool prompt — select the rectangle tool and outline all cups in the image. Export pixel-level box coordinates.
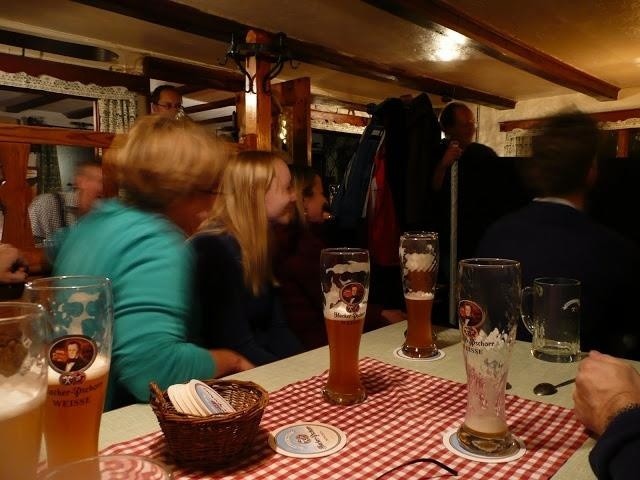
[0,301,48,480]
[318,246,371,404]
[458,257,522,454]
[520,277,582,363]
[24,274,114,480]
[399,231,440,358]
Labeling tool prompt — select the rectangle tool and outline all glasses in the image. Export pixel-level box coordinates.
[154,103,182,110]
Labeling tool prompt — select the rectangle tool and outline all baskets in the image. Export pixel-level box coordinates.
[148,378,269,468]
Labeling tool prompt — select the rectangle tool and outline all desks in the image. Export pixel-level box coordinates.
[1,315,638,480]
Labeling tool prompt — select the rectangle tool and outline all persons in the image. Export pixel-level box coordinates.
[44,110,258,413]
[0,243,31,284]
[431,99,503,329]
[26,155,105,248]
[148,84,182,117]
[570,348,640,480]
[263,164,379,354]
[477,108,639,358]
[185,150,304,368]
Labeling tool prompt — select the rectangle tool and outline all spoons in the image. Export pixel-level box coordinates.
[533,378,576,397]
